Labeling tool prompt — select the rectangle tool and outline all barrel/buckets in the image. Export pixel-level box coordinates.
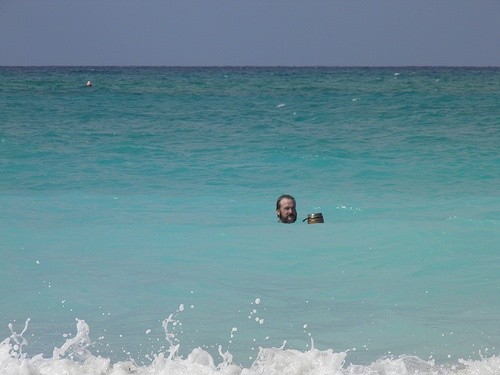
[307,213,324,224]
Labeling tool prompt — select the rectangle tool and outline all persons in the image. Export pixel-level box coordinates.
[274,194,297,224]
